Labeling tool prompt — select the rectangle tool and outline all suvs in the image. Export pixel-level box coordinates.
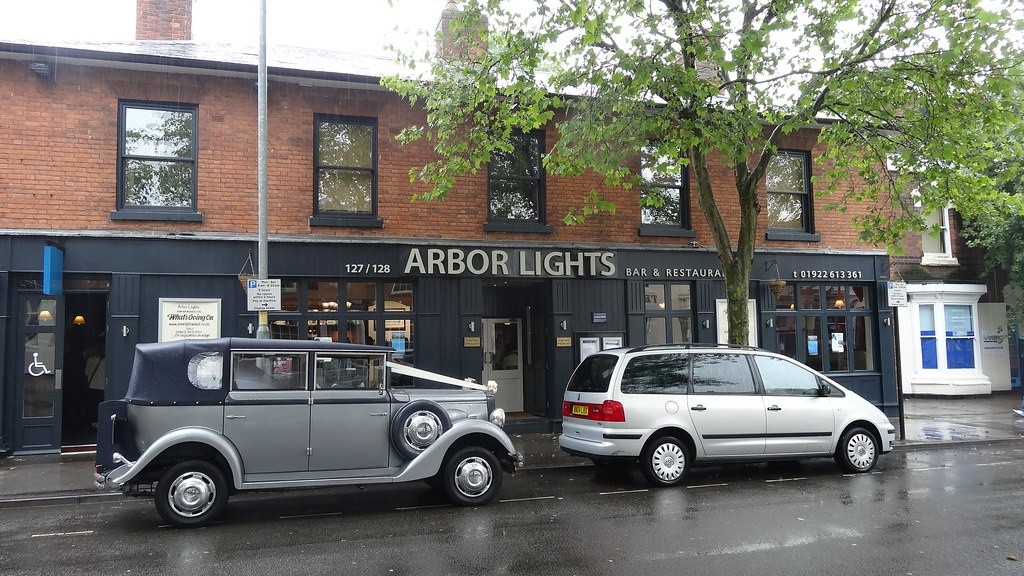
[93,336,526,529]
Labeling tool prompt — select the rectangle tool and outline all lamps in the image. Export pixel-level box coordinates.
[834,299,845,308]
[308,282,352,312]
[38,311,53,321]
[73,316,86,326]
[659,303,665,309]
[790,304,794,310]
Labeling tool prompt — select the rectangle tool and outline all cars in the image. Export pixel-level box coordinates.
[558,343,896,487]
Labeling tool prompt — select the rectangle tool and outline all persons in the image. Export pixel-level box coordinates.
[74,345,106,429]
